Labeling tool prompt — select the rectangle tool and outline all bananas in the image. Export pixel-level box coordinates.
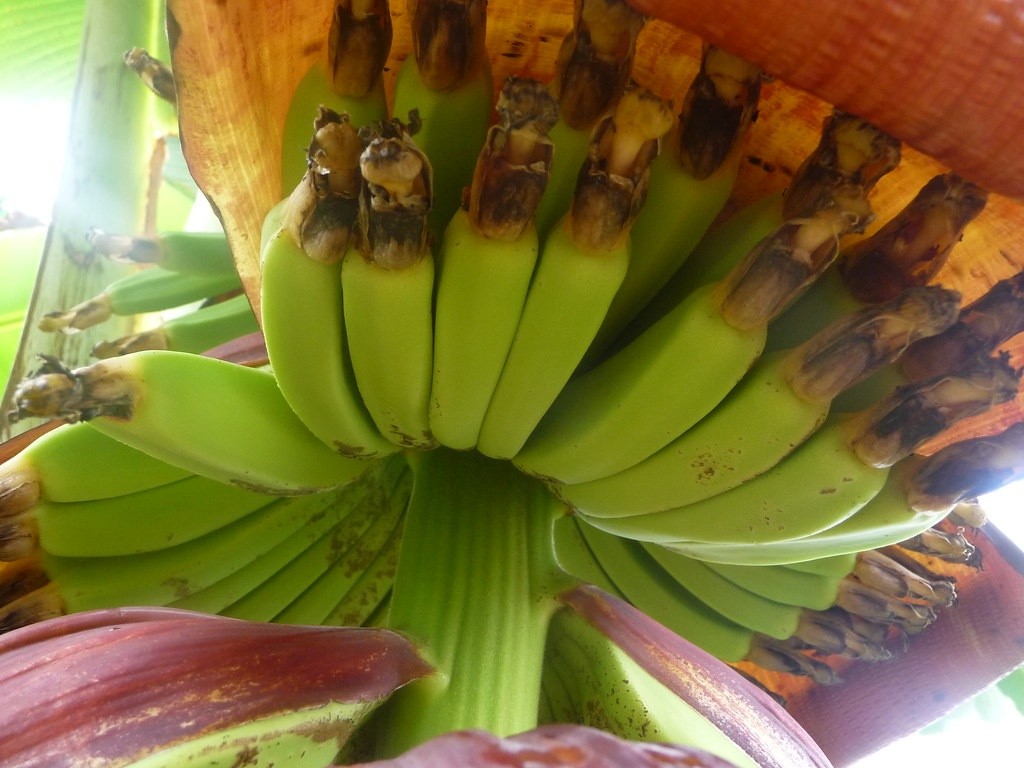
[4,2,1024,768]
